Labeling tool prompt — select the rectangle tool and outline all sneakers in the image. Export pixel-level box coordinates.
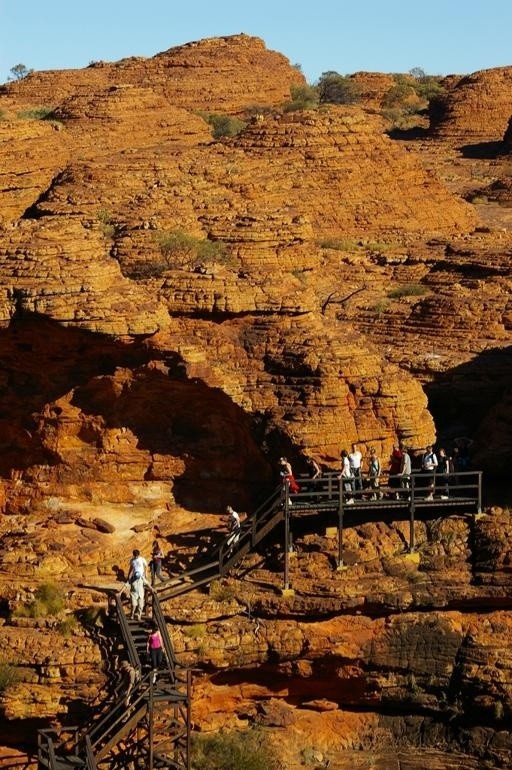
[130,614,141,621]
[345,493,412,504]
[423,494,448,501]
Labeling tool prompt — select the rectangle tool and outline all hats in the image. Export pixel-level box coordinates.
[119,659,131,668]
[134,571,139,577]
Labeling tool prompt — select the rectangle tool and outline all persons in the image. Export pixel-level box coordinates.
[306,434,475,505]
[145,623,164,670]
[119,660,137,725]
[220,504,240,557]
[116,572,157,620]
[150,541,168,581]
[278,456,298,505]
[126,550,148,581]
